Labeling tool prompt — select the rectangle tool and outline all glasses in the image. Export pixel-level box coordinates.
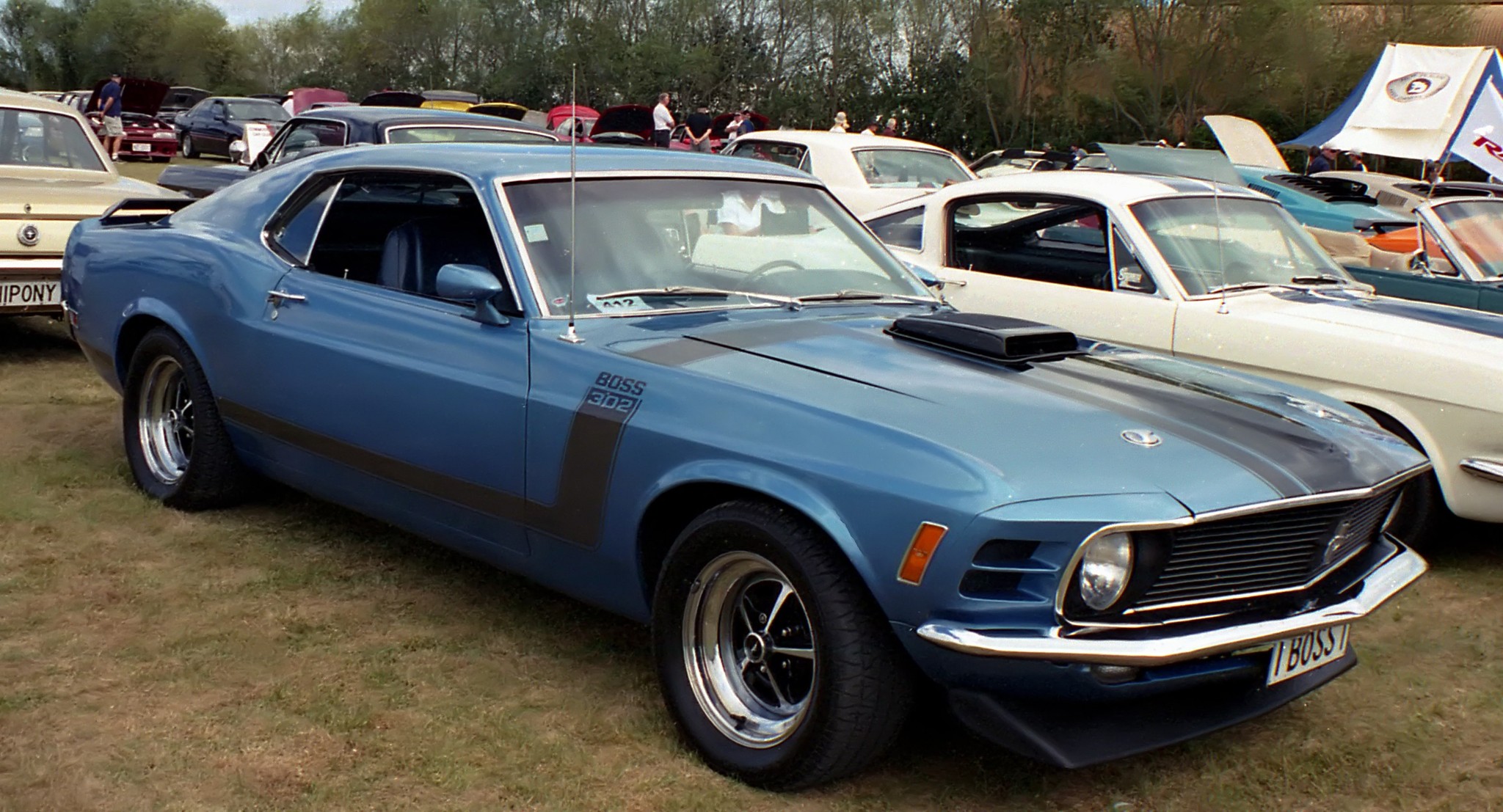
[1324,148,1337,153]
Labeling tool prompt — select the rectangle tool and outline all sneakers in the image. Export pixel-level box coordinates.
[111,157,127,164]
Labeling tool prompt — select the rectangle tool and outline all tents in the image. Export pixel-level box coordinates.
[1275,42,1503,200]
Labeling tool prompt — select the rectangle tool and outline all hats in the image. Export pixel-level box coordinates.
[698,102,707,107]
[1178,142,1187,147]
[1158,139,1166,144]
[734,112,741,115]
[111,73,122,77]
[741,110,750,116]
[834,112,847,123]
[1041,143,1051,150]
[1344,148,1363,157]
[1322,143,1342,155]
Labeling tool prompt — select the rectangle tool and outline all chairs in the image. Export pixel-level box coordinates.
[377,227,513,313]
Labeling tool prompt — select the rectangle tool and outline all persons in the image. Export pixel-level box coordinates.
[737,110,754,138]
[1306,145,1332,175]
[1344,151,1368,172]
[861,120,878,135]
[882,118,897,138]
[1426,161,1444,184]
[684,104,713,155]
[97,73,123,164]
[1042,136,1189,161]
[716,186,765,235]
[653,93,675,148]
[724,112,744,145]
[828,111,847,133]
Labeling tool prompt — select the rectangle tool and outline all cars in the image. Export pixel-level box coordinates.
[28,75,1503,318]
[691,171,1503,552]
[63,144,1431,791]
[0,86,191,345]
